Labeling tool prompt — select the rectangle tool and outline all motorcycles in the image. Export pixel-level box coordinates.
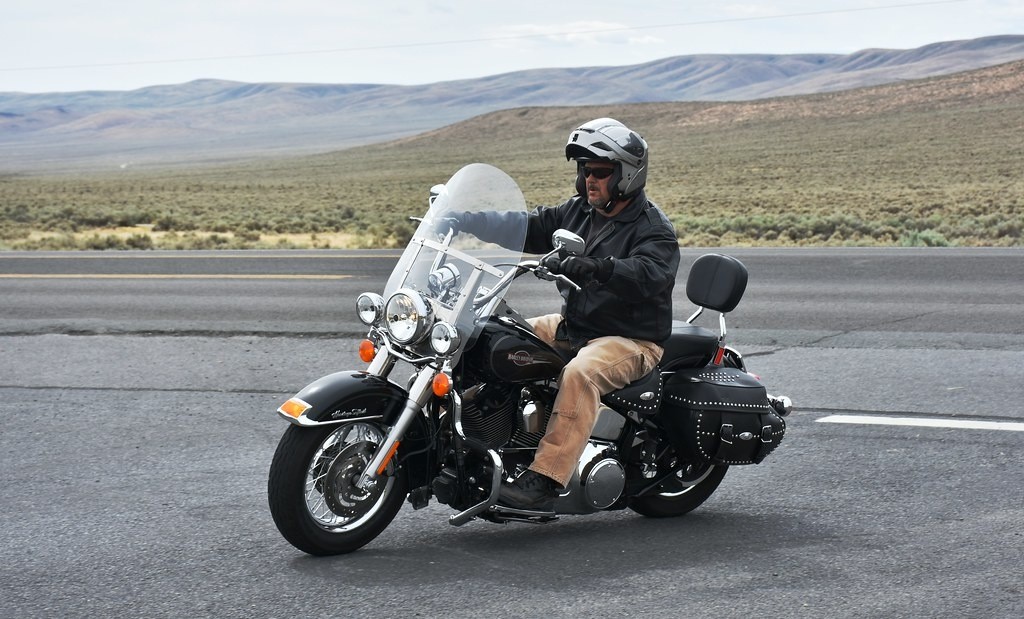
[267,164,792,559]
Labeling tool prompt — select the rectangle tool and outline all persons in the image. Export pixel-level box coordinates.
[435,117,680,516]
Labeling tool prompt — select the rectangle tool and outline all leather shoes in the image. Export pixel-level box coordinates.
[498,470,557,512]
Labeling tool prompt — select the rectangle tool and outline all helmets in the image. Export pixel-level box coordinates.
[565,118,648,213]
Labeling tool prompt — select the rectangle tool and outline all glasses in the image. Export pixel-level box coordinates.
[580,164,615,180]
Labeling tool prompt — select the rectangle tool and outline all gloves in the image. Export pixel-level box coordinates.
[435,208,466,239]
[555,256,599,292]
